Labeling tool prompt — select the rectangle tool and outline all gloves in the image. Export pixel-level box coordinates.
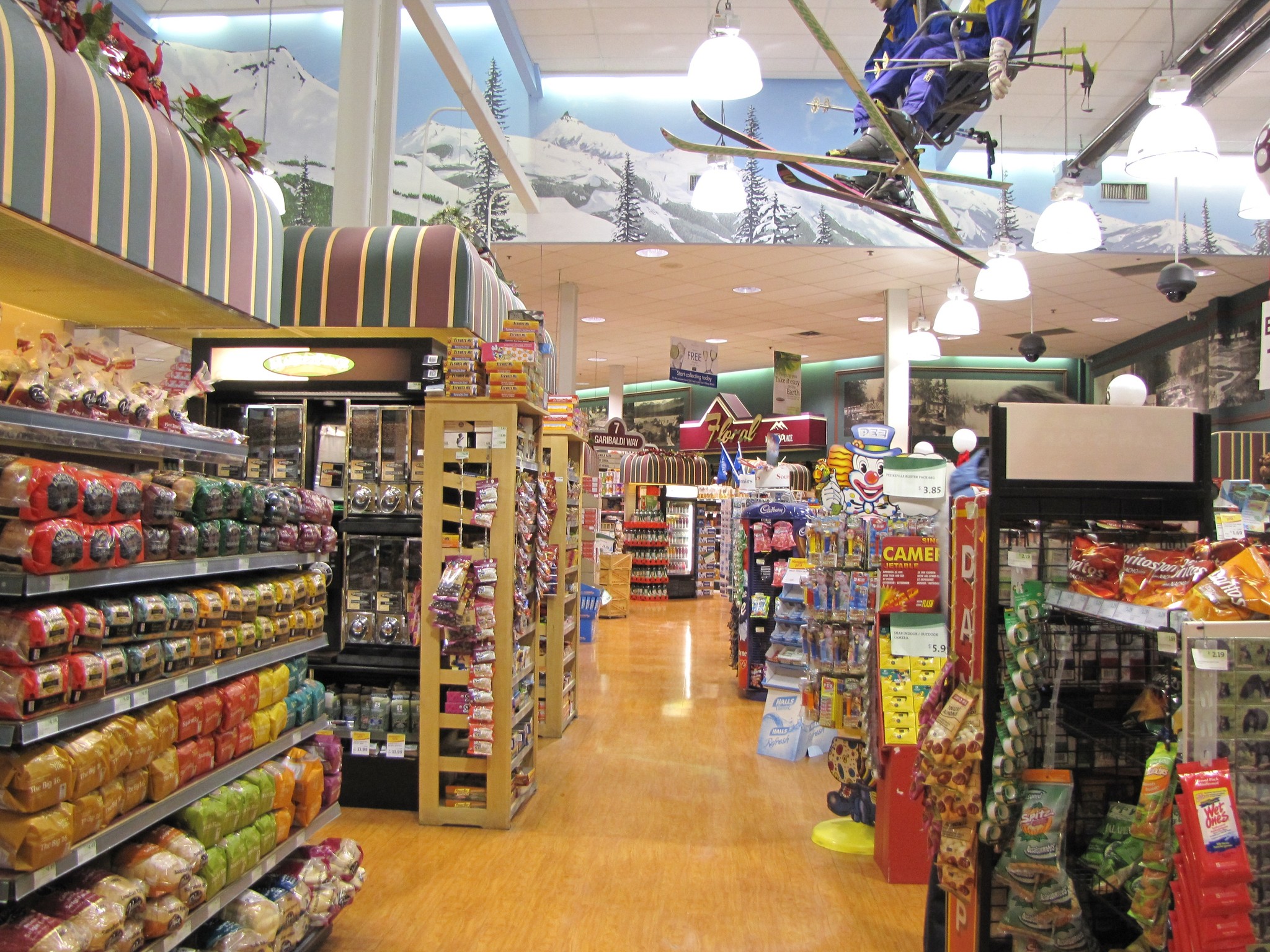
[988,36,1013,100]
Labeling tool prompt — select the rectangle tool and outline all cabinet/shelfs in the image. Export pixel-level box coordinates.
[623,522,669,601]
[419,395,550,828]
[757,560,835,760]
[808,567,877,854]
[0,207,341,952]
[697,504,722,598]
[539,430,587,739]
[188,337,449,808]
[600,495,624,517]
[946,402,1270,949]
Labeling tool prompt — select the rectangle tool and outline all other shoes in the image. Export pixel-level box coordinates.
[880,177,911,207]
[852,171,883,191]
[873,98,924,154]
[841,122,898,165]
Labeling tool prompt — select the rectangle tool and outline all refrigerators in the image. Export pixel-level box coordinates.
[636,485,699,597]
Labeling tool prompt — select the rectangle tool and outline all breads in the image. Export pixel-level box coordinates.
[0,826,369,952]
[0,455,325,722]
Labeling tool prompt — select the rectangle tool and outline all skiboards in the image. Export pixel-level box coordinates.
[660,0,1013,269]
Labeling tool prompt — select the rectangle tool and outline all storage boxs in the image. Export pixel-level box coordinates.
[440,532,535,810]
[821,677,845,729]
[879,637,947,744]
[217,405,424,742]
[598,555,632,616]
[442,314,544,403]
[766,602,807,666]
[539,476,603,724]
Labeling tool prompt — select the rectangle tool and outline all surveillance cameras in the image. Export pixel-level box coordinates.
[1156,263,1197,304]
[1018,333,1046,363]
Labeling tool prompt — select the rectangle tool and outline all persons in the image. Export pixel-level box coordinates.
[833,0,960,211]
[824,0,1024,158]
[924,387,1076,952]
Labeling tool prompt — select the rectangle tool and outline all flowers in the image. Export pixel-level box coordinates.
[39,0,269,174]
[639,449,705,459]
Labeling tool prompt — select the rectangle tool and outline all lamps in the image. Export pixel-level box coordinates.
[1122,0,1217,179]
[687,0,762,211]
[901,0,1270,361]
[249,0,287,217]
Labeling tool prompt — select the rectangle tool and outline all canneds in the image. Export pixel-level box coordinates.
[673,506,687,513]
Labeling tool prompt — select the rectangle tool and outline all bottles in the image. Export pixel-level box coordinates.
[630,583,667,598]
[631,510,664,523]
[631,565,667,579]
[625,547,668,561]
[624,529,669,543]
[666,500,689,574]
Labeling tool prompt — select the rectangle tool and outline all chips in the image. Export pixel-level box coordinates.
[1070,575,1209,622]
